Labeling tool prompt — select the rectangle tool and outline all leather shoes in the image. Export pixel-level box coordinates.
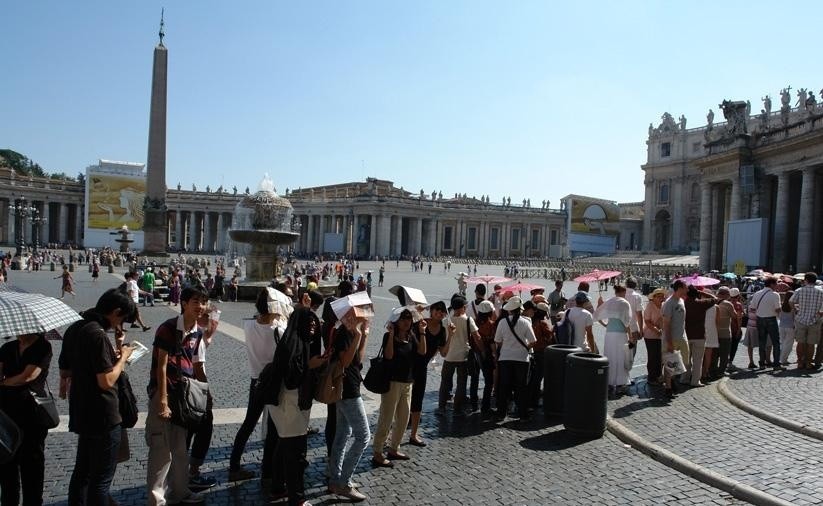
[372,438,426,466]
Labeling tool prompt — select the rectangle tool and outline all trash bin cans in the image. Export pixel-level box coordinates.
[543,344,584,425]
[562,351,610,440]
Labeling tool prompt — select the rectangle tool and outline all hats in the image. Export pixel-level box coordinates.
[649,289,668,299]
[503,296,525,311]
[452,297,468,309]
[730,288,739,297]
[478,300,495,313]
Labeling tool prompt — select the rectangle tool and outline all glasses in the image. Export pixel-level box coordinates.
[402,316,411,320]
[436,307,445,312]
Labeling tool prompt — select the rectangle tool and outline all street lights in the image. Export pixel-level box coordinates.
[5,195,35,271]
[27,207,48,259]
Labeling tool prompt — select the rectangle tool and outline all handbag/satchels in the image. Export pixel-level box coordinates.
[315,328,343,404]
[663,350,687,377]
[468,349,477,376]
[179,376,209,426]
[30,393,59,429]
[117,371,138,428]
[364,344,391,393]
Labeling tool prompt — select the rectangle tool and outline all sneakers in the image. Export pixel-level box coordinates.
[749,361,786,370]
[182,475,216,503]
[680,378,705,387]
[262,487,313,506]
[131,324,150,331]
[439,398,523,421]
[330,480,365,500]
[798,363,815,369]
[230,470,254,482]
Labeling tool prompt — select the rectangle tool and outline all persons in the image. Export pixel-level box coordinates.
[592,285,636,400]
[643,268,823,399]
[598,276,644,386]
[1,245,598,505]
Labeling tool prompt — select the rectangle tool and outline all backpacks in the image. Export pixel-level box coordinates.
[555,309,574,345]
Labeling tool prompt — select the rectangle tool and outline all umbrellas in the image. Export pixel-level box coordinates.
[571,267,622,297]
